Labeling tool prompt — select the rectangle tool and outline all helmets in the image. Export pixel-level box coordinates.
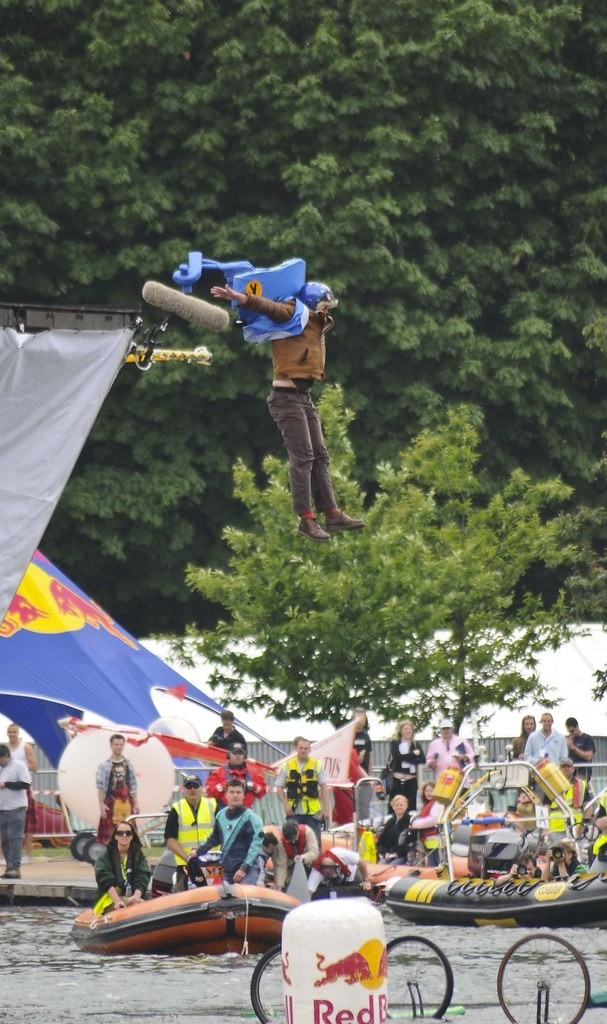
[300,283,335,310]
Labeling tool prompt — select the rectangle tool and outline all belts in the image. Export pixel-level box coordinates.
[273,387,311,394]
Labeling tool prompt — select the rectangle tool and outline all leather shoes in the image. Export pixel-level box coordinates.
[298,516,332,541]
[325,511,365,531]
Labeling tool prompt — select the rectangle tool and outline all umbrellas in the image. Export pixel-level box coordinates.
[0,550,290,787]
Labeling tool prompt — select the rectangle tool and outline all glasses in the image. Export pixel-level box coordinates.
[231,751,243,755]
[185,783,199,789]
[116,831,132,836]
[569,729,574,732]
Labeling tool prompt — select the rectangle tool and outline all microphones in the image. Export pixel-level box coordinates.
[141,282,229,333]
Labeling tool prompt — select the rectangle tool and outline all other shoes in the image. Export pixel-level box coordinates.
[0,868,21,878]
[21,854,32,864]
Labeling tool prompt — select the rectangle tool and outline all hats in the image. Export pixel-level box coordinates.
[440,719,452,728]
[560,758,573,767]
[229,742,246,754]
[183,774,202,787]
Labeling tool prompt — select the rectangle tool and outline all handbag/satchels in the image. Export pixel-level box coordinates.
[397,818,418,846]
[93,883,122,915]
[377,767,395,793]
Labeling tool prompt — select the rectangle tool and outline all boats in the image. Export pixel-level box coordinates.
[384,875,606,927]
[68,883,303,956]
[364,855,554,900]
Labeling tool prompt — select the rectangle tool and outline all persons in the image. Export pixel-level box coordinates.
[89,707,377,906]
[208,282,365,544]
[0,725,43,885]
[370,711,607,893]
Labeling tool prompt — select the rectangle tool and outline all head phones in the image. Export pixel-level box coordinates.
[319,863,341,873]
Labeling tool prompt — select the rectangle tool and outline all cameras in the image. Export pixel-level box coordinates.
[550,846,565,859]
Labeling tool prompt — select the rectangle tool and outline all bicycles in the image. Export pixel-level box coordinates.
[496,933,591,1024]
[250,935,455,1024]
